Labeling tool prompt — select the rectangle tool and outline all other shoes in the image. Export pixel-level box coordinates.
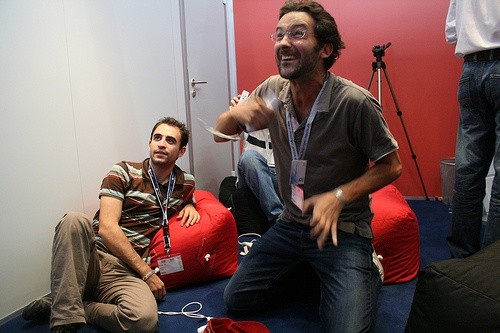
[53,325,77,333]
[23,297,52,321]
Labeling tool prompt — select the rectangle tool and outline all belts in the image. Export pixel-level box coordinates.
[464,50,500,63]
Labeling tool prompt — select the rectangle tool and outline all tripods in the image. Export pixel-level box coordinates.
[367,62,428,201]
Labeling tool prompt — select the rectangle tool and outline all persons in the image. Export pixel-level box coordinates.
[445,0,500,260]
[21,117,200,333]
[213,1,403,333]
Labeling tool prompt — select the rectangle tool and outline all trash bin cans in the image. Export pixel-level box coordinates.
[440,159,457,207]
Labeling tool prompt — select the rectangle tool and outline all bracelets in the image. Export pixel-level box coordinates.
[142,270,156,281]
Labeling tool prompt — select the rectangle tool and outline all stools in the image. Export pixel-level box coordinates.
[404,240,500,333]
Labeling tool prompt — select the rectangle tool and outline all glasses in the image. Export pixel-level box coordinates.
[271,25,319,43]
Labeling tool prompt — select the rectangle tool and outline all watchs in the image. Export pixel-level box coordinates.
[333,187,346,205]
